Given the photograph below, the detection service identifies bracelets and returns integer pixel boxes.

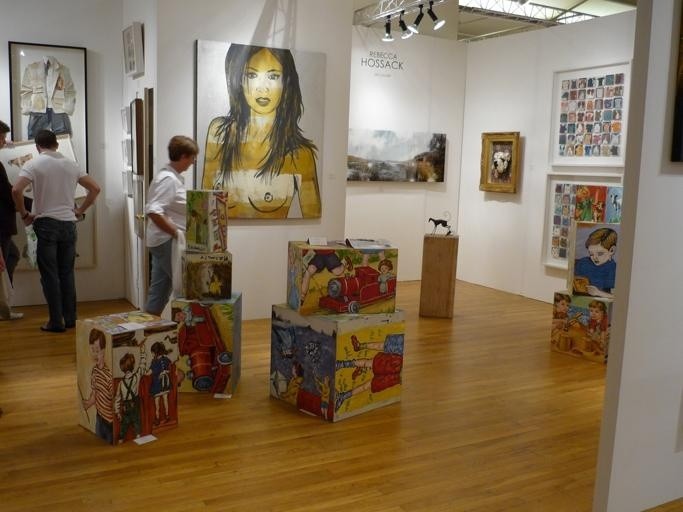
[22,212,29,220]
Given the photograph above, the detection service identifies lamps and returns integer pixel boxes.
[377,1,444,44]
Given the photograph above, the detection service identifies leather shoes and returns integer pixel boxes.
[40,322,65,334]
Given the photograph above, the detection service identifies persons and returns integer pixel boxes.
[301,249,396,306]
[551,292,571,334]
[12,130,100,333]
[335,334,404,411]
[170,306,206,328]
[313,374,331,420]
[81,328,175,445]
[588,300,610,343]
[201,43,321,219]
[0,120,24,321]
[144,136,199,317]
[573,227,618,298]
[278,359,307,406]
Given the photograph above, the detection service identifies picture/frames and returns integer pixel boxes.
[475,129,520,196]
[5,39,88,200]
[118,21,152,241]
[539,171,624,279]
[548,60,634,173]
[4,201,99,273]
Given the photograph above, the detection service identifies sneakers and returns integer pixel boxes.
[3,310,24,320]
